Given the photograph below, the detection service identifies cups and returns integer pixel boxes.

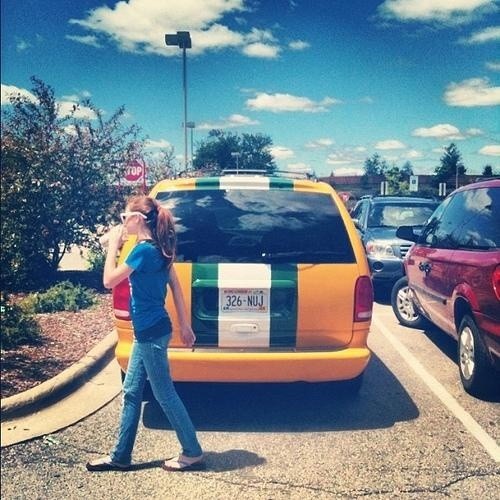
[99,225,120,248]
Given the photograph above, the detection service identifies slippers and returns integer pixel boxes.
[161,458,206,470]
[86,458,130,471]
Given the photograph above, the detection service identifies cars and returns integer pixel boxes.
[109,176,375,394]
[344,182,499,396]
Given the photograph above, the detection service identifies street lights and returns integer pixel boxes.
[165,31,195,177]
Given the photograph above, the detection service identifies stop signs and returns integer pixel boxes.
[123,161,143,182]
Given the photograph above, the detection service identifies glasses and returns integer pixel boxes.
[120,212,148,223]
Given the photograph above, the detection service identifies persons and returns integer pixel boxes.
[86,195,206,472]
